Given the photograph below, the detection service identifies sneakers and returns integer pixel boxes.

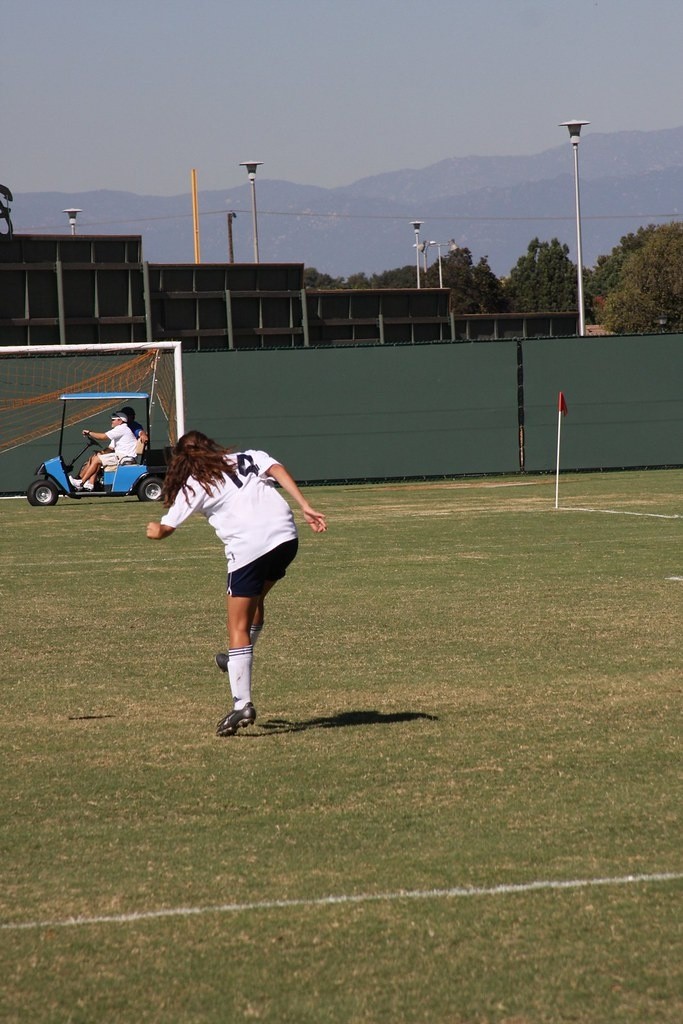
[216,702,255,736]
[215,653,228,671]
[68,475,83,491]
[83,481,94,491]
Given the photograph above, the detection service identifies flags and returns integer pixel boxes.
[558,392,569,417]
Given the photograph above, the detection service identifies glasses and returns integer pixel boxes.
[111,419,115,421]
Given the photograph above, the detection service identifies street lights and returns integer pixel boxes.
[238,160,265,264]
[407,220,424,290]
[412,240,437,288]
[427,240,458,288]
[60,208,82,235]
[558,119,588,336]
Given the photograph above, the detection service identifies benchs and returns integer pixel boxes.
[104,441,148,486]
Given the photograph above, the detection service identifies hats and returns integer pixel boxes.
[110,413,128,422]
[116,407,136,416]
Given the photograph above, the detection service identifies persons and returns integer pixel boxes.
[68,406,149,492]
[146,430,329,737]
